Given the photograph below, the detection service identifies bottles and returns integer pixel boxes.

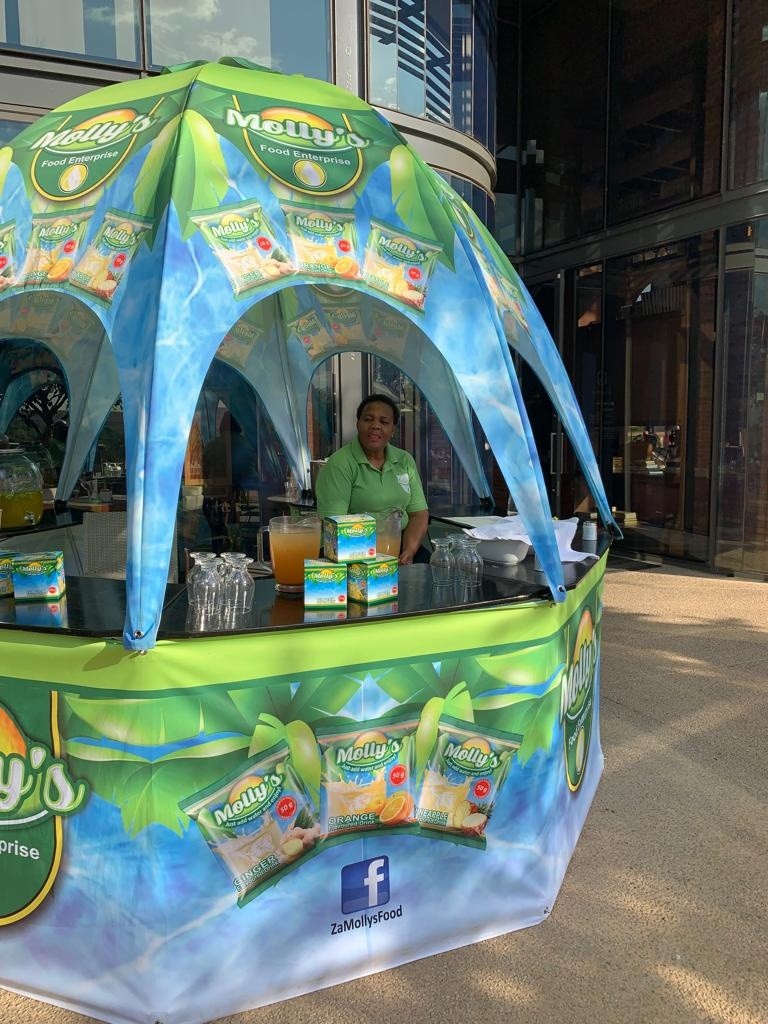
[0,449,43,526]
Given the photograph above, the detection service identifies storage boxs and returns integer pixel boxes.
[0,593,67,628]
[305,601,399,623]
[0,549,66,601]
[323,514,377,563]
[348,553,399,605]
[304,559,347,609]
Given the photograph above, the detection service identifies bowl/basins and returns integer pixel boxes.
[478,540,529,565]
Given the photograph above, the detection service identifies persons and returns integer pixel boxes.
[48,420,68,474]
[315,394,429,565]
[201,411,257,553]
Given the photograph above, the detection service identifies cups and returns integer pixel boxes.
[536,572,548,587]
[582,541,597,554]
[103,462,123,477]
[431,585,484,610]
[534,553,544,572]
[582,521,597,541]
[429,533,484,587]
[184,606,254,634]
[189,551,254,616]
[284,481,295,498]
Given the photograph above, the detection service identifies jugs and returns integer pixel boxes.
[256,516,322,593]
[376,518,401,558]
